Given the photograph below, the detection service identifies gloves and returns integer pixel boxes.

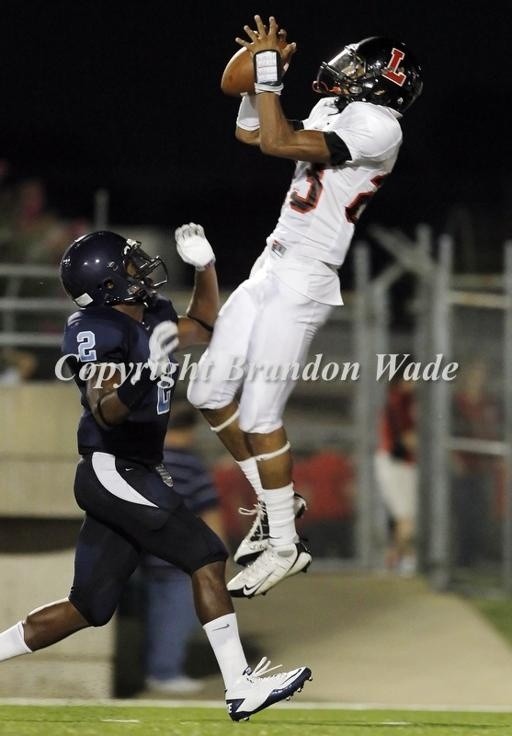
[147,320,179,380]
[174,221,216,272]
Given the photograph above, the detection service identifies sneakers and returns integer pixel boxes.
[234,491,309,567]
[225,539,313,597]
[225,656,313,723]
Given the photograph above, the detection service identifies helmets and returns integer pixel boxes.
[59,230,169,310]
[312,34,424,111]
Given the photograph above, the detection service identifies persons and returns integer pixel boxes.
[184,13,428,596]
[372,353,510,578]
[1,223,313,723]
[1,180,182,384]
[141,397,231,692]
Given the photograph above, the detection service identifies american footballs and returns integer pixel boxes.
[220,40,289,93]
